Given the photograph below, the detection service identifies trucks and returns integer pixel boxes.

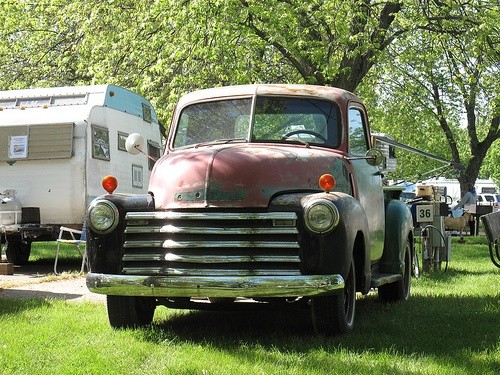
[0,84,164,270]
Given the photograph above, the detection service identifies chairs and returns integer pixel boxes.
[53,220,92,275]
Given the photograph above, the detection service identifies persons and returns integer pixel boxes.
[454,184,479,236]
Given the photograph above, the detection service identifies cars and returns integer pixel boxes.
[82,82,416,339]
[449,193,500,215]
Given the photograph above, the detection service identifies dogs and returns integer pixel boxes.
[444,211,473,242]
